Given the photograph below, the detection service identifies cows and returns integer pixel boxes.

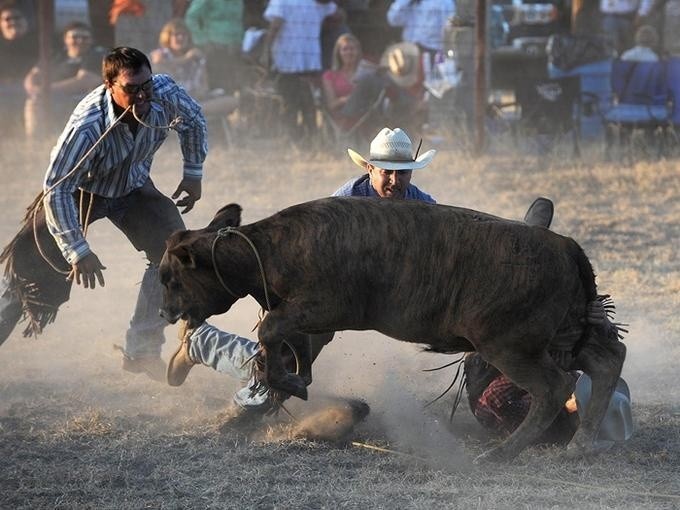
[155,194,627,465]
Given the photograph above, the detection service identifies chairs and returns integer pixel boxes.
[198,54,680,167]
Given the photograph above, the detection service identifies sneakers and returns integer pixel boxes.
[166,318,201,387]
[121,353,169,381]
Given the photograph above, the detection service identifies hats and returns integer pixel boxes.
[377,40,424,90]
[574,368,637,446]
[346,126,438,172]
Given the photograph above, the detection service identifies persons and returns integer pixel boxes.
[463,351,634,446]
[164,126,438,414]
[1,45,207,379]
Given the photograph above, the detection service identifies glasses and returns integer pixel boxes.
[110,76,154,95]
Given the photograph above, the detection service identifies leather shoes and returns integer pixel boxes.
[522,196,555,230]
